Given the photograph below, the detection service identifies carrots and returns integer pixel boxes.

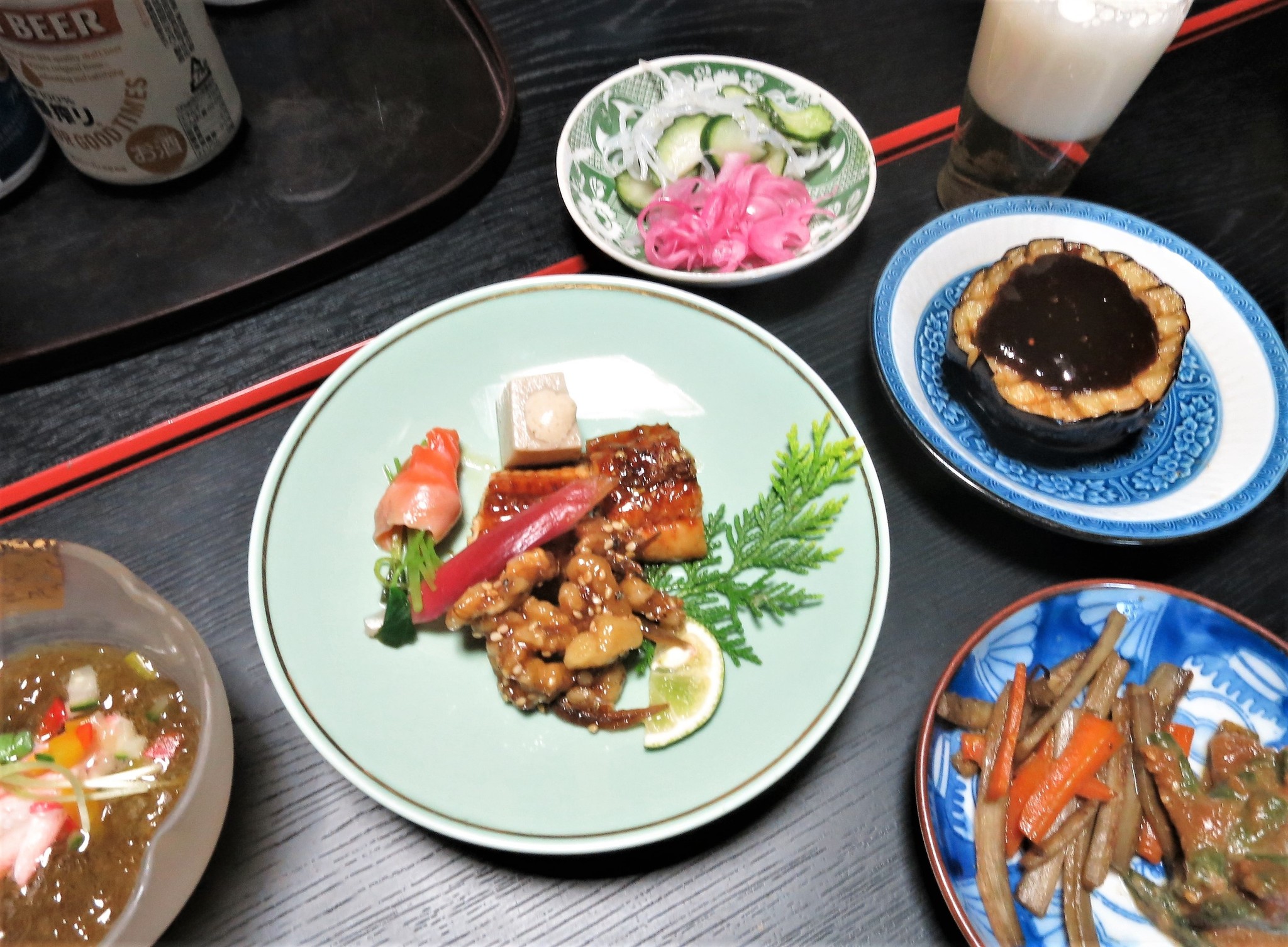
[959,662,1194,862]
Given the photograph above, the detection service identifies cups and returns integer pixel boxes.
[936,1,1192,212]
[0,0,245,185]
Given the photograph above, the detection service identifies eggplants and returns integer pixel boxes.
[943,238,1191,449]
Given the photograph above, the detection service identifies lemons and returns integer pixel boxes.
[643,616,724,750]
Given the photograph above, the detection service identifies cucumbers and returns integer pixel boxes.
[615,87,833,214]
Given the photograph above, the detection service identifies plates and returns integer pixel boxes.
[0,58,48,197]
[555,51,879,289]
[868,194,1288,544]
[249,276,892,854]
[913,577,1288,947]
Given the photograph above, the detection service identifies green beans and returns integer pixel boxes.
[1123,729,1288,947]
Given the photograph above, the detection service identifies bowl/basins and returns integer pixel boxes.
[0,539,235,947]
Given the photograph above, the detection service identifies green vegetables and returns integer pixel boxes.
[372,438,445,648]
